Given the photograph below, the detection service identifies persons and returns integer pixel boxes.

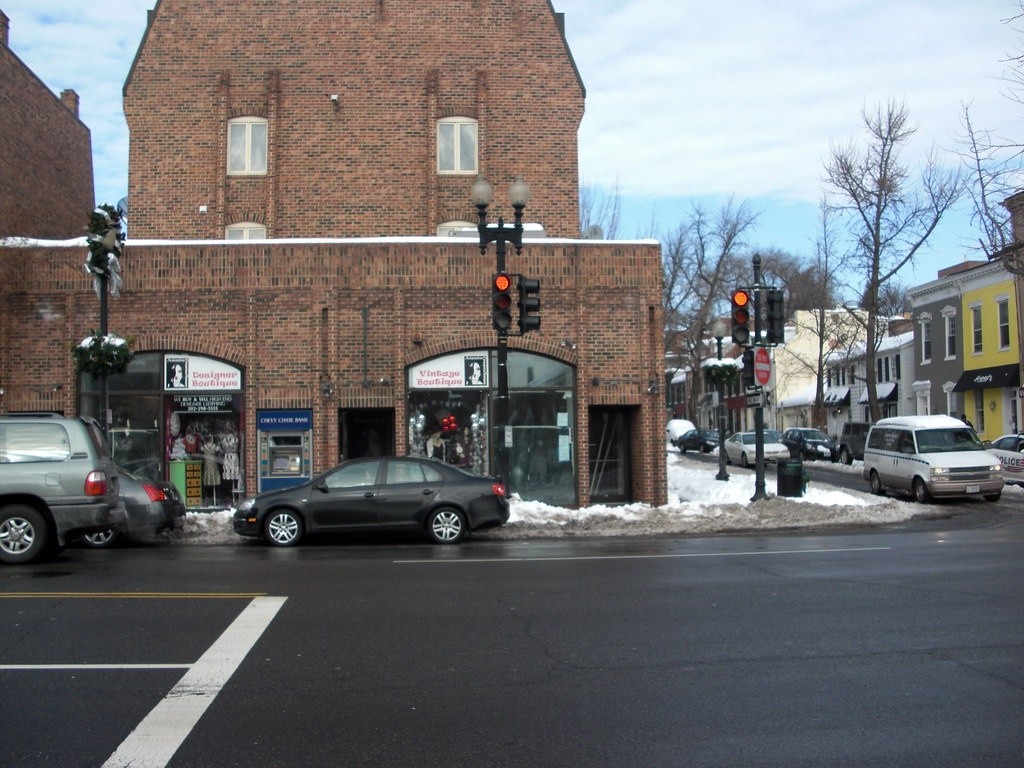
[183,419,240,484]
[426,426,472,467]
[961,414,976,433]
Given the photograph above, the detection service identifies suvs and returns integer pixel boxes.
[0,414,125,565]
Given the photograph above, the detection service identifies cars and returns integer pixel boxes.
[666,419,694,445]
[778,427,838,463]
[984,434,1024,487]
[676,429,720,454]
[76,464,187,551]
[233,455,512,548]
[721,431,791,464]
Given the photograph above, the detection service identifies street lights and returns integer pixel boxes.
[712,318,731,480]
[467,173,530,508]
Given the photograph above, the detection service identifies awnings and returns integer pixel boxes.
[951,363,1021,392]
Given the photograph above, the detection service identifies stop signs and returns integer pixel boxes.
[754,348,772,384]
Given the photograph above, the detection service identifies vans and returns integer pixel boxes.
[863,414,1004,504]
[838,421,876,464]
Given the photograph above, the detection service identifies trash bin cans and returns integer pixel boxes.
[777,459,804,497]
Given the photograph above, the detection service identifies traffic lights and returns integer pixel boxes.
[520,272,542,332]
[492,274,513,334]
[730,289,752,346]
